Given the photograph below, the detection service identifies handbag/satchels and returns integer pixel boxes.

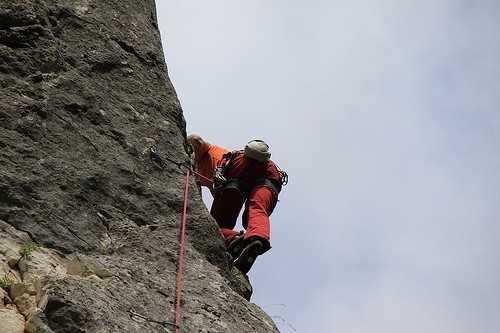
[244,140,271,161]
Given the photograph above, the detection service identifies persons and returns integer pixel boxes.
[187,134,282,275]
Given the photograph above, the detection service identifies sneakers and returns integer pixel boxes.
[225,233,247,260]
[233,240,262,276]
